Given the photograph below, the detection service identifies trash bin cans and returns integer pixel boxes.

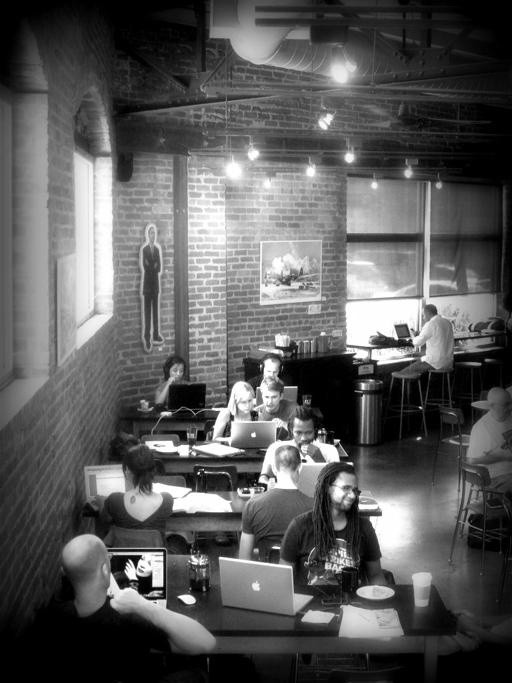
[354,379,385,445]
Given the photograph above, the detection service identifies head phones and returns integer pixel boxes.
[256,353,284,374]
[164,356,180,373]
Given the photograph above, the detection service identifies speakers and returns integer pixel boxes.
[117,151,133,182]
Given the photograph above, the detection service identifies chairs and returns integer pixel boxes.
[445,464,511,578]
[431,403,474,494]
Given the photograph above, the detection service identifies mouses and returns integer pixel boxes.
[176,593,197,605]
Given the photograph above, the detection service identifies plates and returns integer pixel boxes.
[355,584,396,601]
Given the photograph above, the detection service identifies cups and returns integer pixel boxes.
[341,565,357,589]
[303,394,312,405]
[186,427,198,448]
[413,571,432,605]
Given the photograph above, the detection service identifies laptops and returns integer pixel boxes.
[107,547,168,609]
[228,420,277,449]
[296,462,329,498]
[256,385,298,407]
[164,383,206,414]
[83,463,132,501]
[394,324,411,342]
[218,556,315,617]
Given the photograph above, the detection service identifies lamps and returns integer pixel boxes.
[218,46,450,197]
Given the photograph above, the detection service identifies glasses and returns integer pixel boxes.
[330,484,361,496]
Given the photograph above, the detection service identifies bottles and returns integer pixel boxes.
[186,550,211,588]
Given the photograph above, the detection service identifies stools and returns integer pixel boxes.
[346,334,511,433]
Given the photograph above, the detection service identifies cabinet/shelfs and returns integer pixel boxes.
[242,349,358,444]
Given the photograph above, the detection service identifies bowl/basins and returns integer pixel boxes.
[236,485,265,497]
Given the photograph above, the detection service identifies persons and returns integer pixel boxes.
[257,407,340,492]
[437,615,512,682]
[246,354,294,390]
[97,444,172,545]
[144,226,163,349]
[214,381,258,443]
[123,553,155,596]
[237,445,315,562]
[34,532,215,655]
[154,355,190,405]
[277,462,387,585]
[464,386,512,502]
[412,304,453,376]
[257,376,298,423]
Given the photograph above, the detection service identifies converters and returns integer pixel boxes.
[159,411,173,417]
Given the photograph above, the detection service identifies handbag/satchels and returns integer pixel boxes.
[108,432,140,457]
[369,332,398,346]
[467,513,512,552]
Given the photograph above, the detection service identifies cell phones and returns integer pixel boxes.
[411,329,415,333]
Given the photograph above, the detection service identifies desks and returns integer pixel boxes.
[161,581,459,682]
